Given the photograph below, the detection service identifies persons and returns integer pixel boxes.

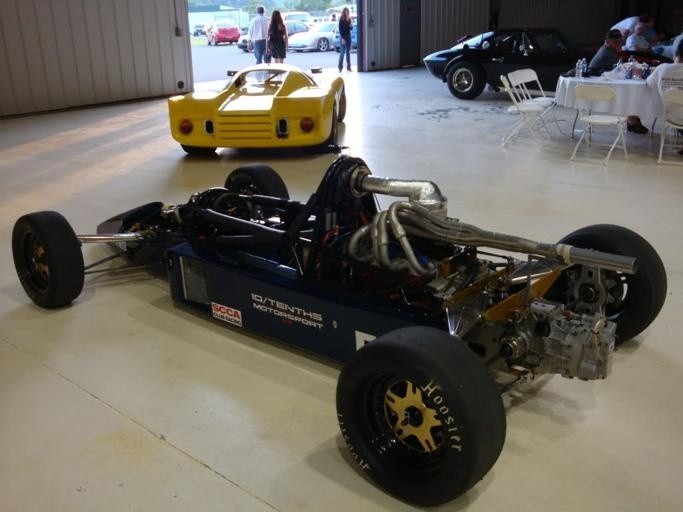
[331,14,337,22]
[264,11,288,63]
[246,6,271,63]
[338,7,353,72]
[586,15,683,160]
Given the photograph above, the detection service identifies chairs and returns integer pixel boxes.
[506,68,566,135]
[657,86,682,165]
[498,75,553,149]
[570,84,626,165]
[267,154,377,277]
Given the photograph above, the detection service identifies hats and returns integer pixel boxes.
[605,29,623,39]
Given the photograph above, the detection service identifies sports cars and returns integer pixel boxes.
[163,60,345,159]
[420,23,672,99]
[8,156,669,511]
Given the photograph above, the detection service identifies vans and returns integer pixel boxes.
[280,11,313,26]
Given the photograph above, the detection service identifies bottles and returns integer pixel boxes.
[616,56,647,80]
[575,58,587,78]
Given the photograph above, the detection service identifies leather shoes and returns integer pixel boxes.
[627,119,648,133]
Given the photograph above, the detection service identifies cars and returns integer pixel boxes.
[192,23,206,37]
[234,21,310,55]
[206,19,239,47]
[287,21,339,53]
[333,16,358,52]
